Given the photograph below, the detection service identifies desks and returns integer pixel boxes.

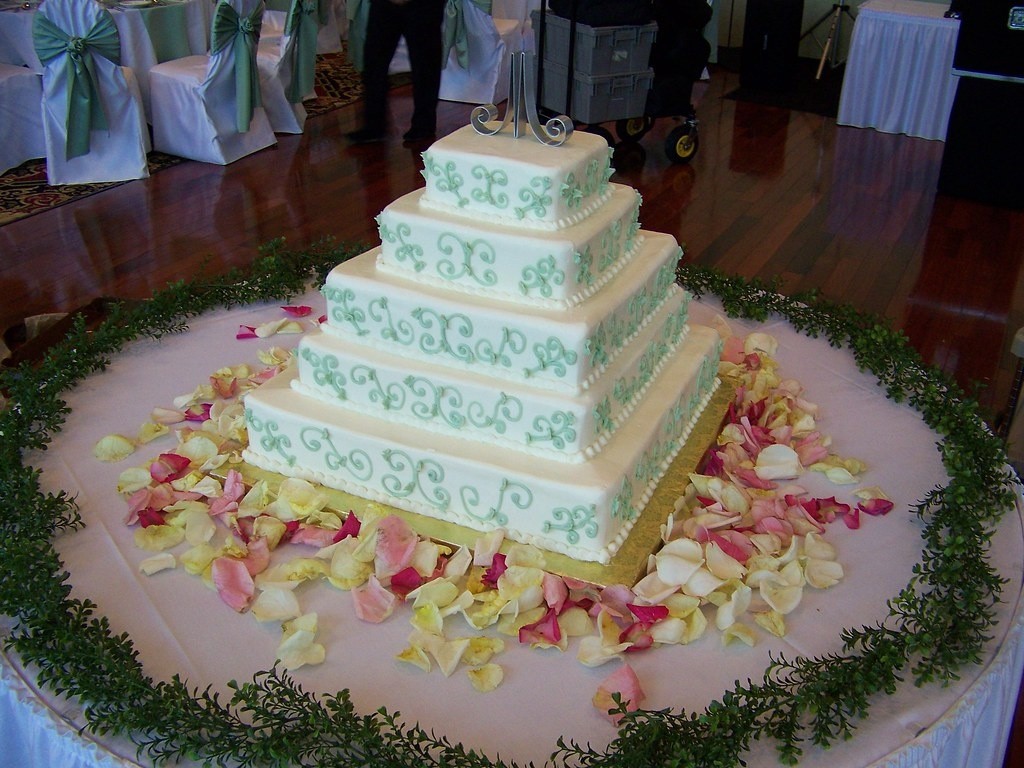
[835,0,962,142]
[0,0,213,128]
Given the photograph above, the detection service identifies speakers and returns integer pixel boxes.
[740,0,804,95]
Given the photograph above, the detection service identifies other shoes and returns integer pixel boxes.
[402,123,437,138]
[345,123,391,140]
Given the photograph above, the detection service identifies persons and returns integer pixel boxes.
[343,0,447,141]
[737,0,806,104]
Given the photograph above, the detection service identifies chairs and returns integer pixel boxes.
[32,0,153,186]
[151,0,279,166]
[346,0,411,76]
[207,0,323,136]
[437,0,524,107]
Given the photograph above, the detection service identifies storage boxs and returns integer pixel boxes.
[529,10,658,76]
[531,54,654,124]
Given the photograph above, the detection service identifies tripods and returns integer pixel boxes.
[800,0,856,81]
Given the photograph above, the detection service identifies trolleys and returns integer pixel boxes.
[531,0,715,165]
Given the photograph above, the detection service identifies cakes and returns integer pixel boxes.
[243,120,722,564]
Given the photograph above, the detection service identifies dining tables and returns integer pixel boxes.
[0,243,1024,768]
